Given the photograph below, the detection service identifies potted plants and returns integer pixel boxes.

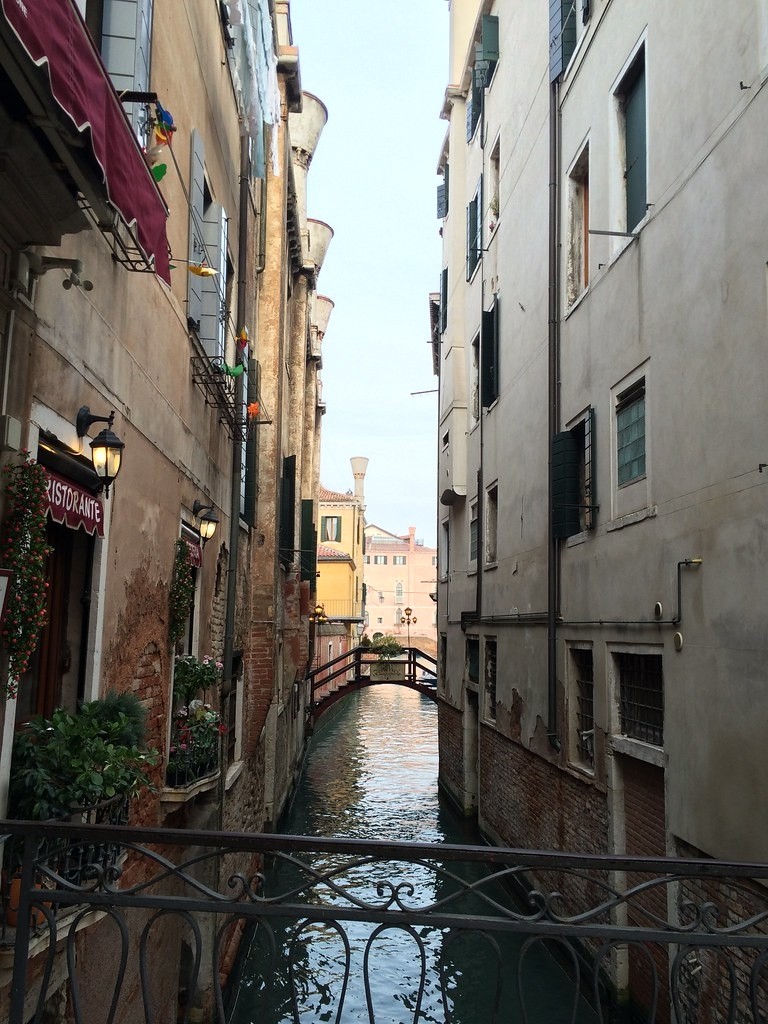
[2,689,157,927]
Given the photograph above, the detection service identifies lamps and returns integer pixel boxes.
[308,605,328,625]
[193,500,220,549]
[76,405,126,500]
[400,607,417,625]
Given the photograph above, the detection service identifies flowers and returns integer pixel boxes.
[167,652,229,785]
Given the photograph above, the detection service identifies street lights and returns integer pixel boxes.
[308,604,329,670]
[400,606,417,681]
